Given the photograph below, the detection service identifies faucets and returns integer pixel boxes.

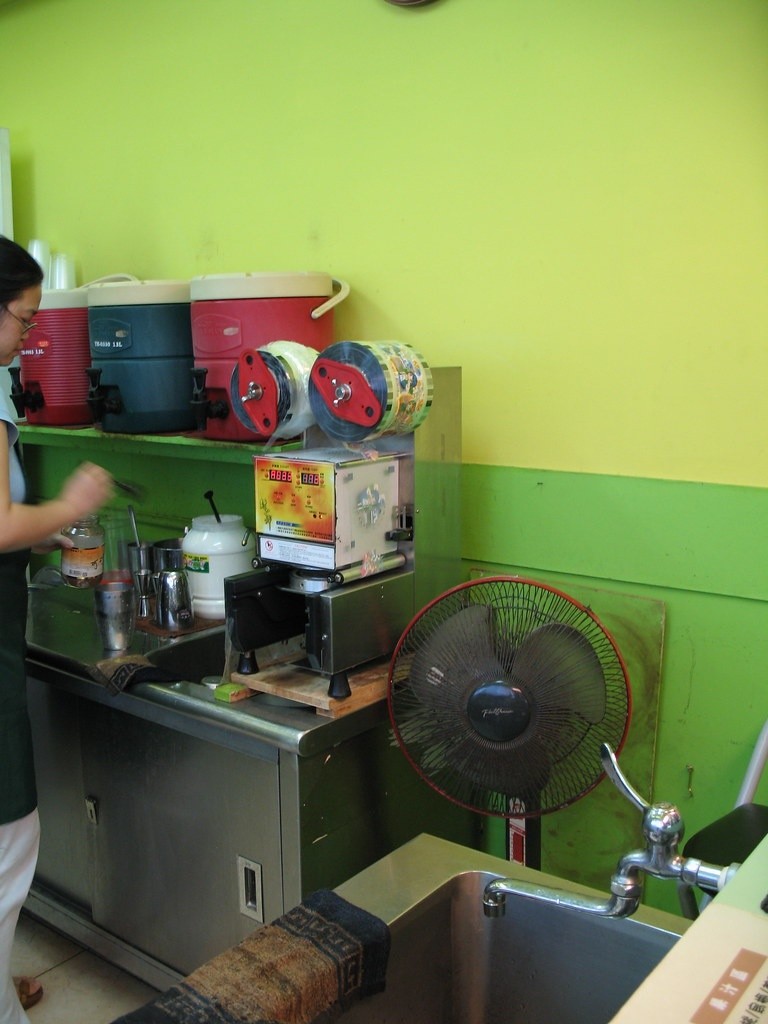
[600,743,738,920]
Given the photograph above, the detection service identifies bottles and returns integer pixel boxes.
[182,513,258,620]
[60,514,105,589]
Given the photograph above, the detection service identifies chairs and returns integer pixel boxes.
[683,717,768,914]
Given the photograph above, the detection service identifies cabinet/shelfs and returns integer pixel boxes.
[22,676,281,995]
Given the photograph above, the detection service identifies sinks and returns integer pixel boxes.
[106,832,699,1024]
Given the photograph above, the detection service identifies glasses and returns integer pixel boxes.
[3,303,38,334]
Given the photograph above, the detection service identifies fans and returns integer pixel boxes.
[386,576,632,870]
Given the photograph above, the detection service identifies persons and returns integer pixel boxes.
[0,236,114,1024]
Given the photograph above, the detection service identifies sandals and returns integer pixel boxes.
[11,974,44,1009]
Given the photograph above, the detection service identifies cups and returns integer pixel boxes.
[94,582,138,651]
[51,252,76,289]
[97,510,143,585]
[28,238,51,290]
[152,537,182,572]
[100,568,134,585]
[151,570,195,630]
[126,541,154,620]
[30,566,70,590]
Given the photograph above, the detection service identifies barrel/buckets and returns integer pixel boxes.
[190,271,353,441]
[7,288,94,425]
[78,273,192,435]
